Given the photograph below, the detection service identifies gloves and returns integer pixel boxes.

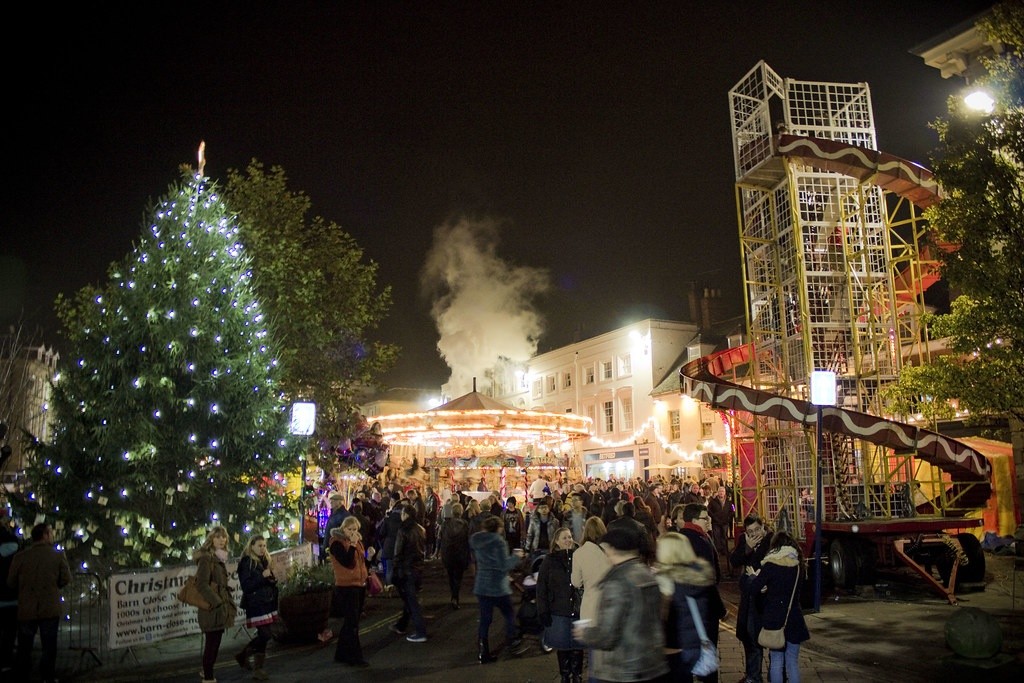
[539,613,553,627]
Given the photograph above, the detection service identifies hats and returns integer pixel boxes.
[330,495,344,500]
[391,493,400,500]
[649,486,658,491]
[508,496,516,505]
[614,500,626,515]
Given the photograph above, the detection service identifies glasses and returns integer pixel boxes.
[698,516,709,521]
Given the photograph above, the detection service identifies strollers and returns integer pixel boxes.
[508,555,553,654]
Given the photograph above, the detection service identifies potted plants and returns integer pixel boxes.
[277,559,333,640]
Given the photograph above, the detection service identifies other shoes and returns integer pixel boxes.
[561,677,570,683]
[199,670,216,683]
[572,675,582,683]
[452,599,462,609]
[335,657,369,668]
[739,675,763,683]
[389,623,406,634]
[406,632,427,642]
[415,585,424,592]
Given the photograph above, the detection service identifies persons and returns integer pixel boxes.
[727,513,811,683]
[407,453,418,475]
[303,477,315,496]
[536,526,584,683]
[178,526,237,683]
[388,504,428,642]
[435,503,475,610]
[322,469,743,542]
[572,529,676,683]
[329,515,371,668]
[655,532,720,683]
[570,516,613,629]
[468,514,531,664]
[8,523,73,683]
[235,535,280,679]
[679,503,731,623]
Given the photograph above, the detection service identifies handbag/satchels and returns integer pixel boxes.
[758,626,786,649]
[180,557,218,610]
[691,641,721,677]
[367,573,383,597]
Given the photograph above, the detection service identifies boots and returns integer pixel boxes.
[509,636,531,655]
[236,647,257,672]
[478,637,498,664]
[430,538,442,560]
[253,654,270,680]
